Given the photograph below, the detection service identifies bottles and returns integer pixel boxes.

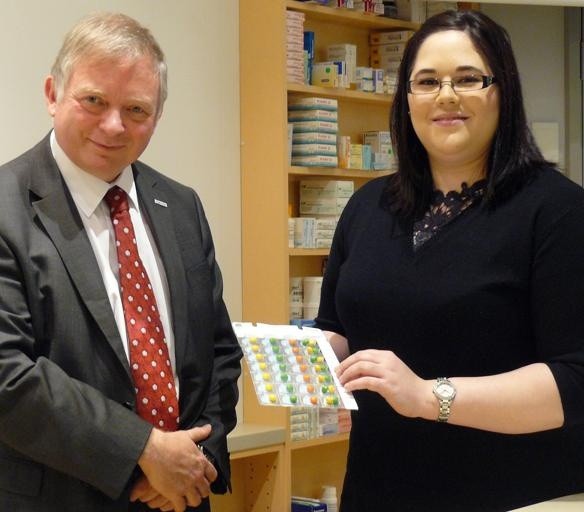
[319,486,337,512]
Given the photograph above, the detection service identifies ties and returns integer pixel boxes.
[105,186,180,432]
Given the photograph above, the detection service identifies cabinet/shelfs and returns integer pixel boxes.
[239,2,483,512]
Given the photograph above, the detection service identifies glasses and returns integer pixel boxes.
[403,74,497,93]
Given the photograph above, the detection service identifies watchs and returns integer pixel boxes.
[433,376,456,424]
[197,445,216,466]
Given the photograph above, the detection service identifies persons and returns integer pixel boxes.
[0,10,243,512]
[313,9,584,512]
